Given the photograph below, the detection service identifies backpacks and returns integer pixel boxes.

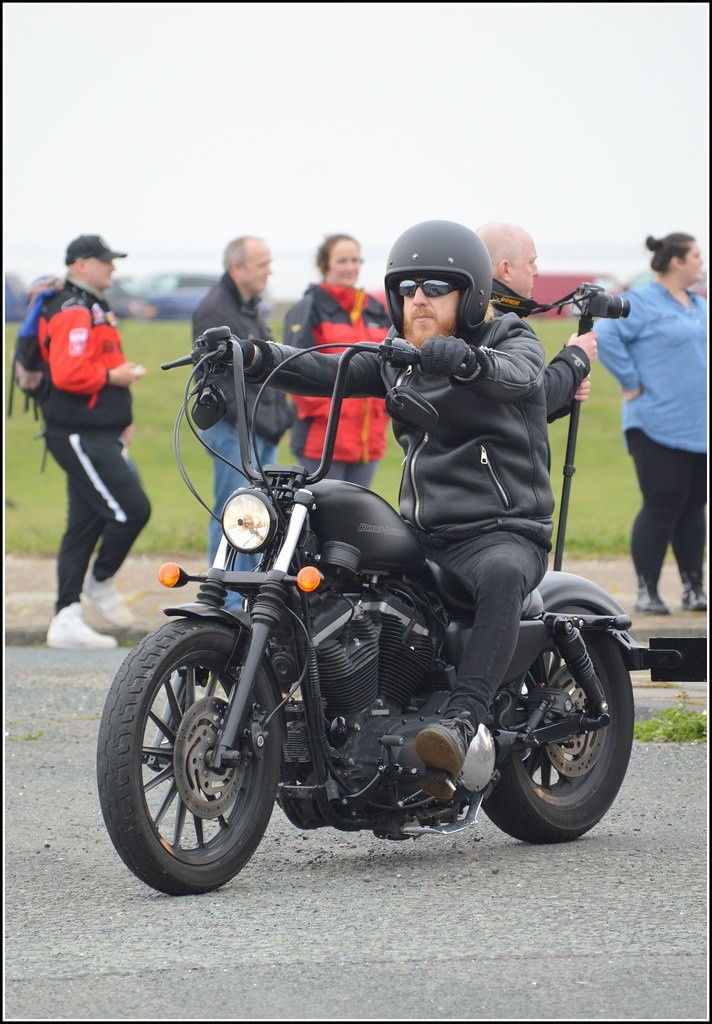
[12,289,94,405]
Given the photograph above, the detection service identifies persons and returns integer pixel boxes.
[192,221,546,799]
[37,226,706,647]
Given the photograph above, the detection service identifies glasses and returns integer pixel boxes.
[398,278,462,298]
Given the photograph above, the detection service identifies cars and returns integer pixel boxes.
[130,273,272,322]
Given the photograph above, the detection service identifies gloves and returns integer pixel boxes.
[192,334,255,374]
[416,335,478,379]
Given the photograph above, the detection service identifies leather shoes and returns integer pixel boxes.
[415,711,475,803]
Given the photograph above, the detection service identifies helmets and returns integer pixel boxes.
[385,220,493,334]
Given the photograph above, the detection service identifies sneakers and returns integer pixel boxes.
[681,584,707,610]
[82,572,135,627]
[46,602,118,651]
[635,587,669,615]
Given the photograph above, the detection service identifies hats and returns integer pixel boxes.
[66,235,127,264]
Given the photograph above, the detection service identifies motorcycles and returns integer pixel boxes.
[96,327,707,894]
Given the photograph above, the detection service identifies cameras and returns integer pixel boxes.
[571,283,630,319]
